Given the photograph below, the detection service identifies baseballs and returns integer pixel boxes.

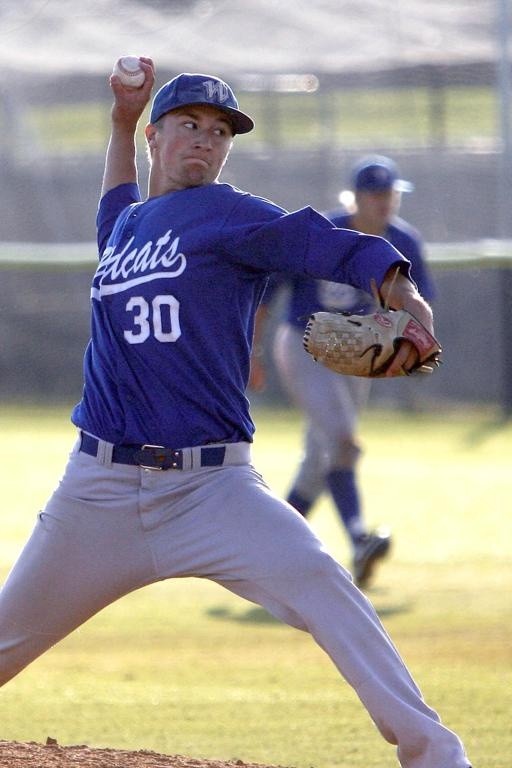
[114,57,149,90]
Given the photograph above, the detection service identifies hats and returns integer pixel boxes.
[150,75,254,136]
[357,165,396,191]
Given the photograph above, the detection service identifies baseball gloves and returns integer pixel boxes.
[303,306,441,379]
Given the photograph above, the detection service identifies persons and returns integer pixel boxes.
[244,154,441,592]
[0,56,471,768]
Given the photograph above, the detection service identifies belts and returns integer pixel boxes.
[79,430,226,469]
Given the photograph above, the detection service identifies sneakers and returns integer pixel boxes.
[353,532,388,585]
